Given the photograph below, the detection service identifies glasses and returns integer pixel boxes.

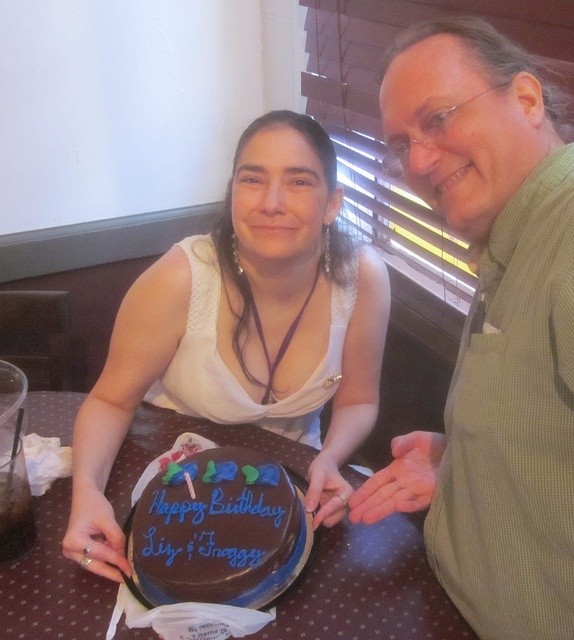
[381,83,510,177]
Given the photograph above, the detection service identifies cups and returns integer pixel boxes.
[1,359,35,560]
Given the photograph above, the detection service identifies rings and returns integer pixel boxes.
[80,560,94,567]
[334,495,349,509]
[81,538,94,557]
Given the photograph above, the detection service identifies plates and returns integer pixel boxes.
[119,466,319,612]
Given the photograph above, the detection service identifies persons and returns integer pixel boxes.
[62,109,390,582]
[348,16,574,640]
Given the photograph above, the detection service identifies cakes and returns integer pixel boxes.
[126,446,306,609]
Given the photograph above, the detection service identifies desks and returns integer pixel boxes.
[0,390,479,640]
